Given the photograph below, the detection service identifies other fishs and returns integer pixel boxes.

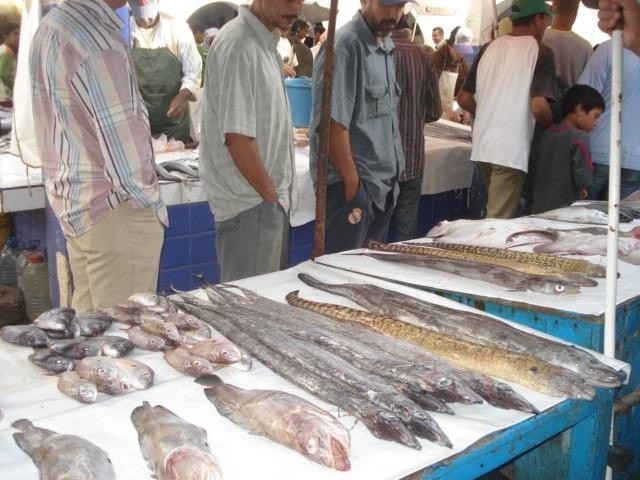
[159,286,422,452]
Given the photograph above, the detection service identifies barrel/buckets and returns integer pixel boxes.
[284,76,312,128]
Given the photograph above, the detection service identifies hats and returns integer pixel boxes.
[127,0,158,19]
[508,0,555,22]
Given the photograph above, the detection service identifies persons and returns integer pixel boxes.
[188,21,210,144]
[432,27,448,50]
[200,0,304,283]
[527,84,606,214]
[310,25,326,60]
[205,27,220,48]
[436,26,473,125]
[0,23,21,108]
[129,0,202,144]
[542,0,594,100]
[577,39,640,201]
[597,0,640,56]
[456,0,558,220]
[307,0,419,255]
[28,0,169,316]
[277,27,299,80]
[387,11,443,242]
[291,19,313,77]
